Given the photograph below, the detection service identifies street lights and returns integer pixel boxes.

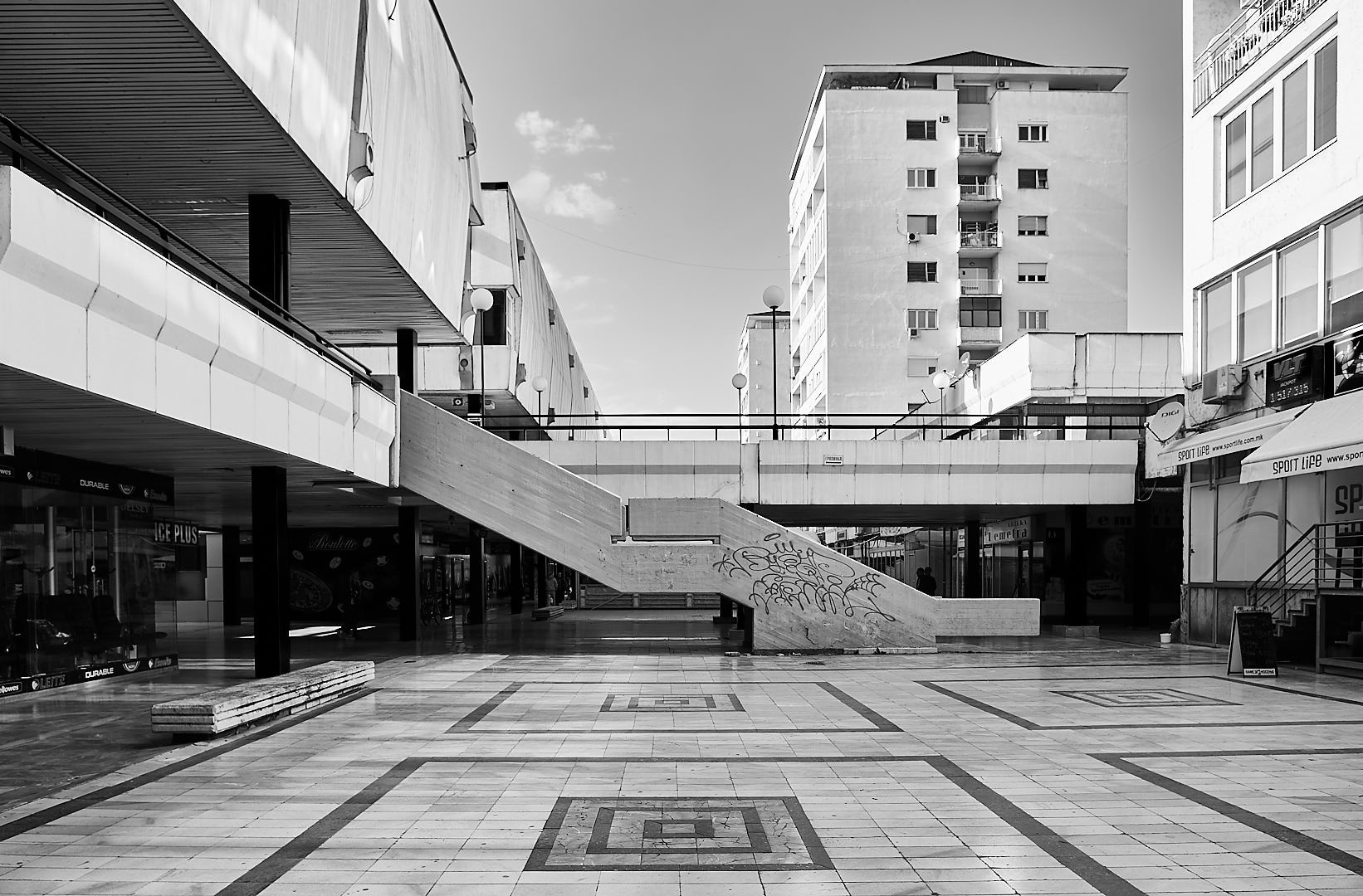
[933,371,951,441]
[763,286,784,440]
[470,286,494,430]
[531,374,546,440]
[731,373,747,442]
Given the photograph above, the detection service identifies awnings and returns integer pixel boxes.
[1157,388,1363,484]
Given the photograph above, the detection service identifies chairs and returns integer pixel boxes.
[12,593,167,667]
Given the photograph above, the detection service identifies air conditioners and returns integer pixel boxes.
[940,116,950,123]
[1202,364,1242,403]
[996,79,1009,90]
[908,328,919,338]
[907,232,920,243]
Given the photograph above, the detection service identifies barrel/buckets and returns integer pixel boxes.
[1160,633,1172,643]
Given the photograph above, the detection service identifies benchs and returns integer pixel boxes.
[532,607,564,620]
[151,661,376,738]
[559,600,577,613]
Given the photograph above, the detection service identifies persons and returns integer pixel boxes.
[916,567,937,597]
[547,571,566,606]
